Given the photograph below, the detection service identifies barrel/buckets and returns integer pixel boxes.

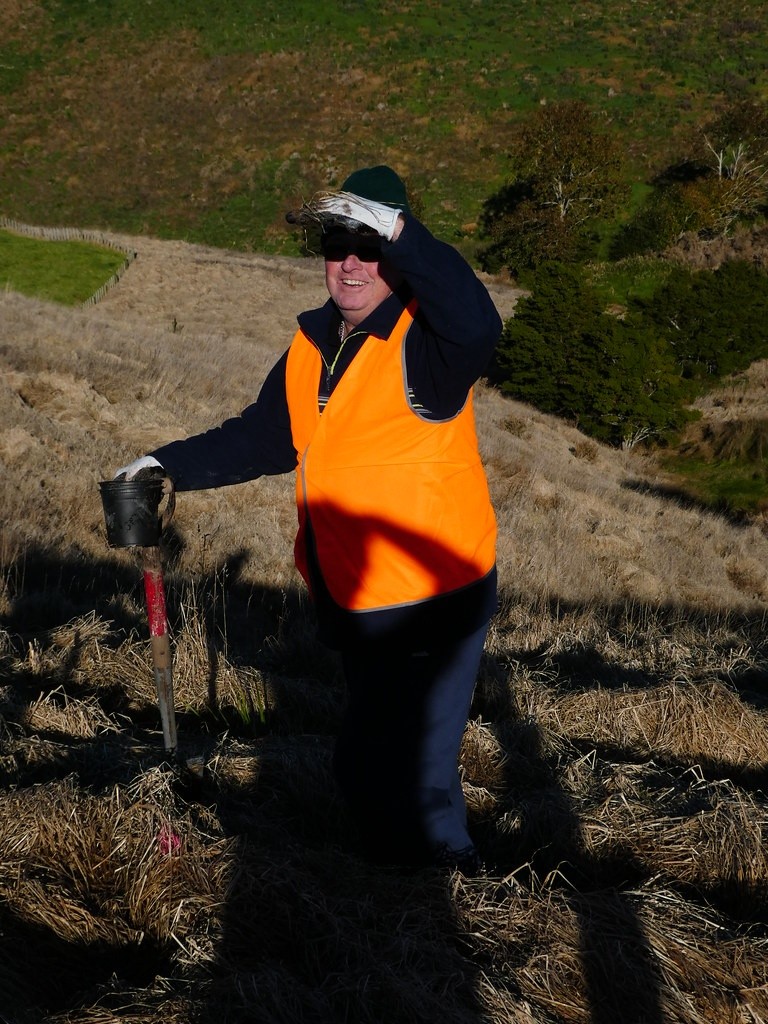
[96,479,165,547]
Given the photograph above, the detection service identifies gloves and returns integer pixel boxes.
[285,190,404,241]
[113,456,165,482]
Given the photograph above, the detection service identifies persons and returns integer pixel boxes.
[115,164,504,895]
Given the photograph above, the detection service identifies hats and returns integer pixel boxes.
[340,164,411,214]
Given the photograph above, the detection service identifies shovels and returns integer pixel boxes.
[107,470,204,797]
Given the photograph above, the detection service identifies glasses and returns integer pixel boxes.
[320,244,385,262]
